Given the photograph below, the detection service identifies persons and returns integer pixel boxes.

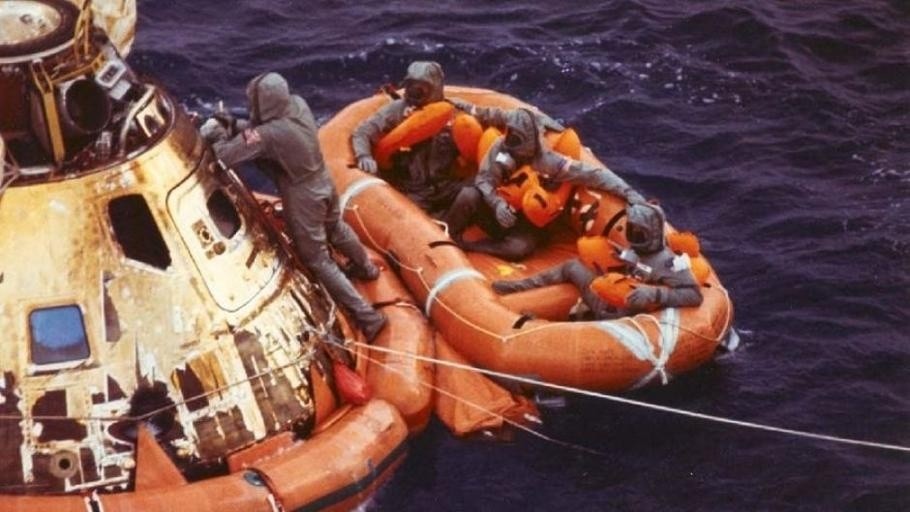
[490,201,703,320]
[202,72,387,344]
[350,62,565,216]
[439,108,647,263]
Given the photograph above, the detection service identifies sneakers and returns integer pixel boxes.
[344,264,379,281]
[364,312,386,342]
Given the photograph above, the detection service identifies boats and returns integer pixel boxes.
[316,86,736,388]
[1,0,436,512]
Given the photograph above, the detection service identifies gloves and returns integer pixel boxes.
[357,155,378,174]
[626,282,668,311]
[495,201,517,229]
[627,190,646,205]
[542,116,565,133]
